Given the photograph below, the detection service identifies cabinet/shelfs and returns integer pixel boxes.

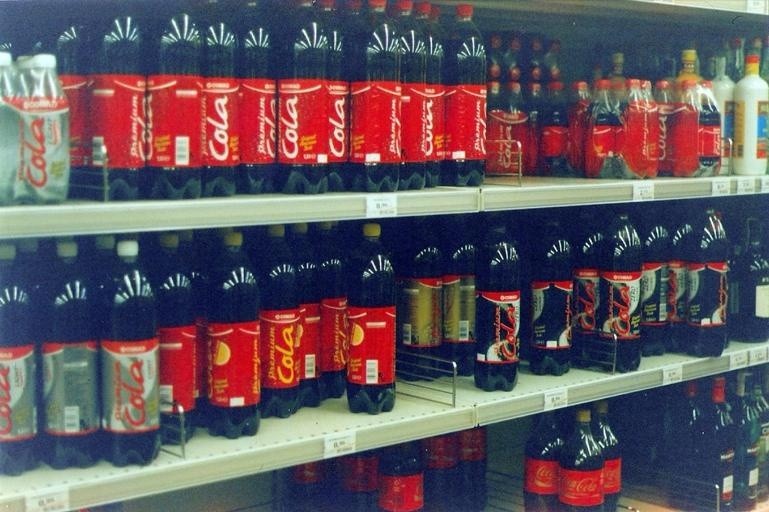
[0,173,768,511]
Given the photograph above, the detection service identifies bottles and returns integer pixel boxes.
[268,369,768,512]
[2,0,769,207]
[2,201,767,480]
